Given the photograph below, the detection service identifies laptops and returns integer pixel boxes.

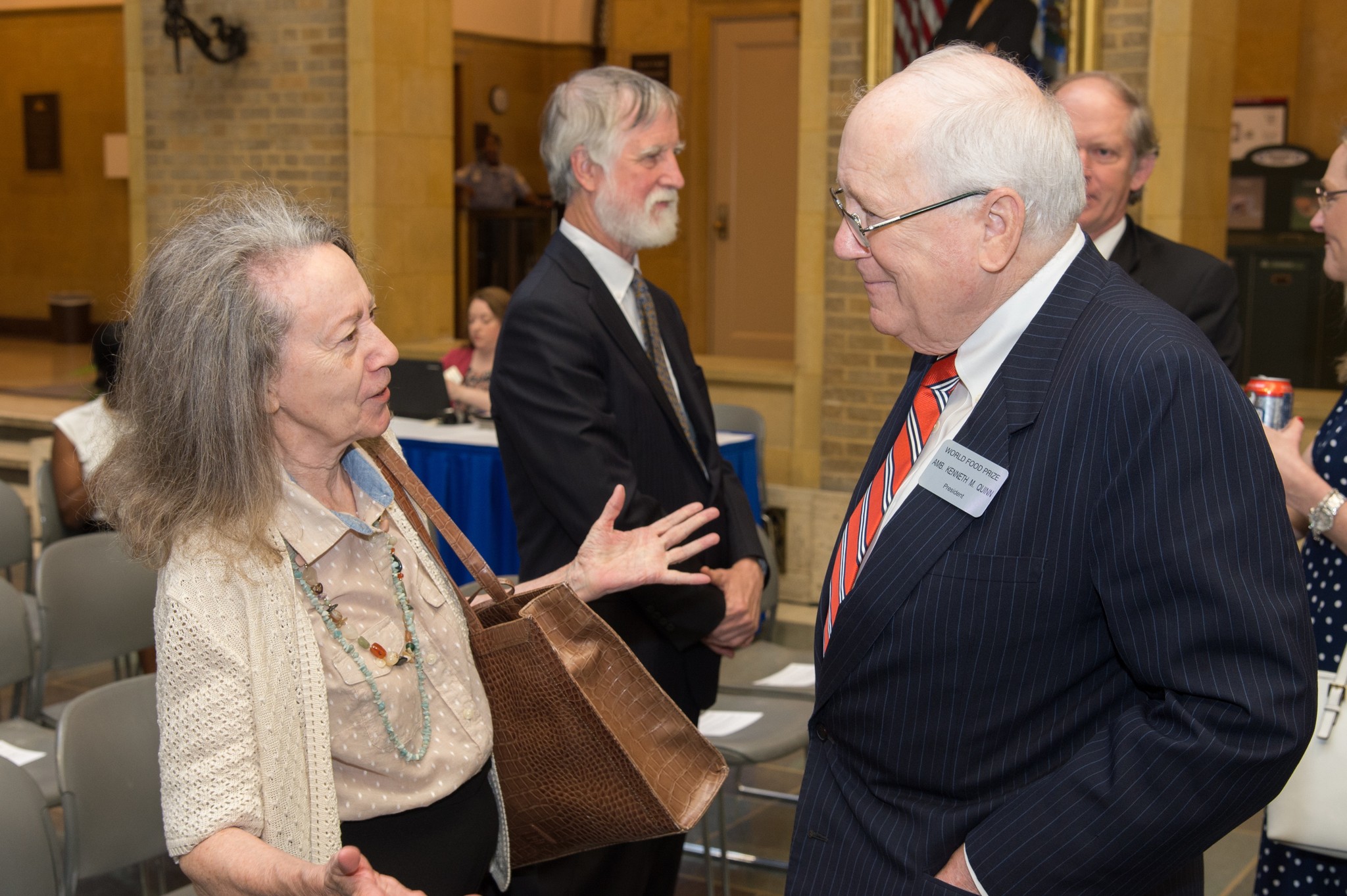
[387,358,461,423]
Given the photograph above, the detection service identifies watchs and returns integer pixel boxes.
[1309,486,1346,543]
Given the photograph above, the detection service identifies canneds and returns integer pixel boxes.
[1244,374,1293,430]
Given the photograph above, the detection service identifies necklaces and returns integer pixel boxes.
[280,514,433,764]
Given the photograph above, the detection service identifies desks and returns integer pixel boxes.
[393,419,762,585]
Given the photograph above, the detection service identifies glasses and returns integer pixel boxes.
[1314,185,1346,211]
[830,188,993,247]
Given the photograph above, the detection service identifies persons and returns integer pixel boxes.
[454,133,551,289]
[1043,69,1248,388]
[785,42,1321,896]
[492,67,773,895]
[1251,142,1347,895]
[91,186,719,896]
[50,323,127,536]
[439,287,511,415]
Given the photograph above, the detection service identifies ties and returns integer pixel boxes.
[822,350,962,657]
[631,268,701,461]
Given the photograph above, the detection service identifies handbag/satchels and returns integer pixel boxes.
[359,432,729,870]
[1263,644,1347,860]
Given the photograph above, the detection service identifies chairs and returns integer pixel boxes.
[0,460,199,896]
[684,406,815,896]
